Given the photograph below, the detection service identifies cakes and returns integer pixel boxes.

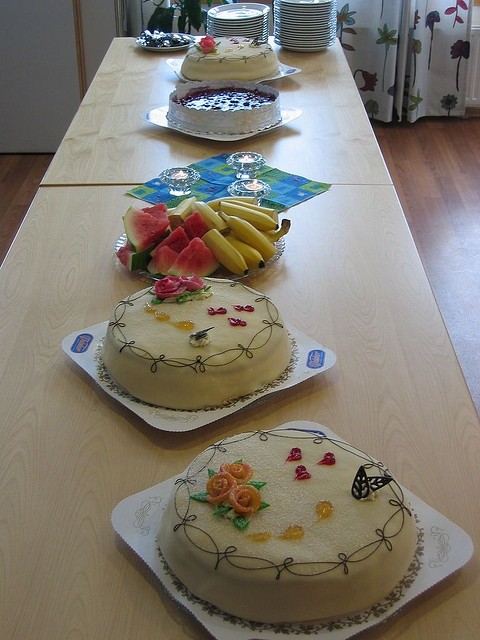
[181,35,280,80]
[166,80,283,135]
[99,275,291,409]
[154,427,419,622]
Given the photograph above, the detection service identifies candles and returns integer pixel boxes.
[225,179,271,207]
[156,166,201,196]
[224,151,268,179]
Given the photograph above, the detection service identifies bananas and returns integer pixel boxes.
[171,196,290,277]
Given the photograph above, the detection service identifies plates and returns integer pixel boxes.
[274,0,337,53]
[134,32,196,52]
[113,207,285,282]
[206,3,270,45]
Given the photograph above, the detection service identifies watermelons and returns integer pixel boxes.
[118,202,219,277]
